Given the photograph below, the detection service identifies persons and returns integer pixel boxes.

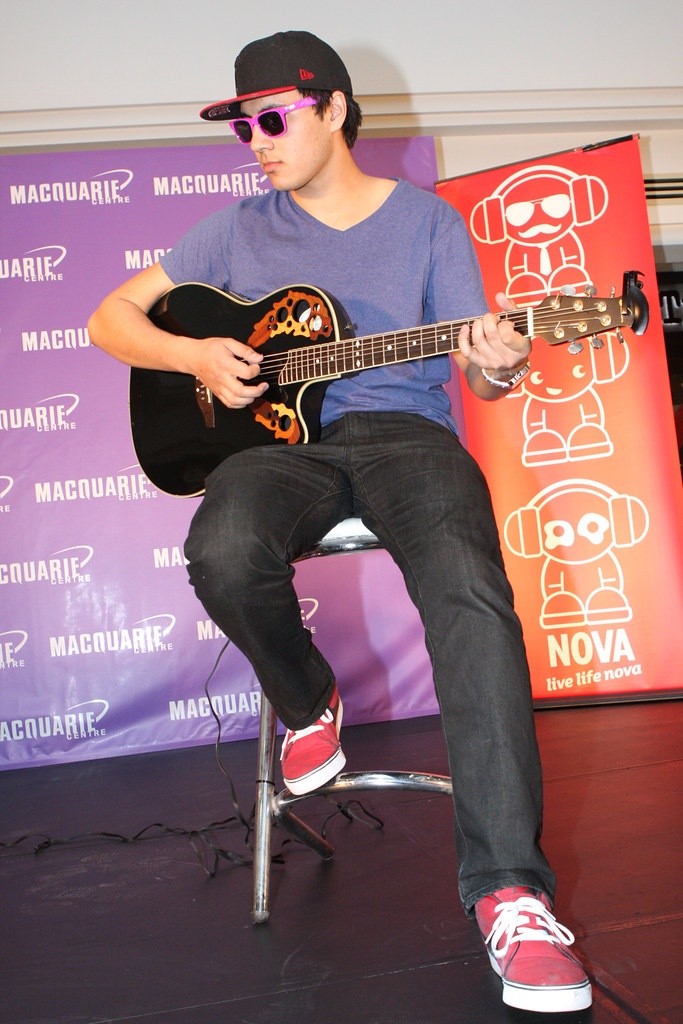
[86,31,593,1012]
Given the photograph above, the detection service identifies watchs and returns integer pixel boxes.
[481,362,532,388]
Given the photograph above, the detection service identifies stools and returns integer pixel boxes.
[250,516,454,922]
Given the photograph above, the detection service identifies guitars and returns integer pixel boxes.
[127,269,651,500]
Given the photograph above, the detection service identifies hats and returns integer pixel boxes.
[198,30,353,120]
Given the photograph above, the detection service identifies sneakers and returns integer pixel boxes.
[279,675,347,796]
[472,885,593,1013]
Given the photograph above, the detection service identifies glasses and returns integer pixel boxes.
[229,94,322,144]
